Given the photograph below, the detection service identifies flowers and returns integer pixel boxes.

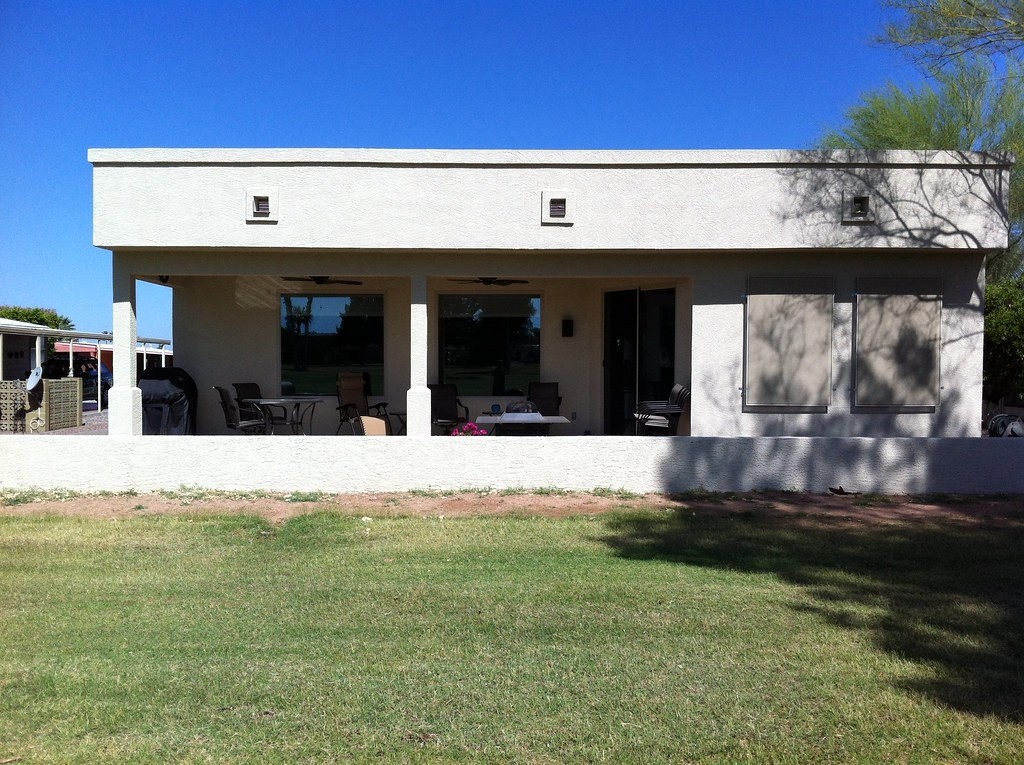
[451,422,487,436]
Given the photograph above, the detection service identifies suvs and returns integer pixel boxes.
[17,355,113,407]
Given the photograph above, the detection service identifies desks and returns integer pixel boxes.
[389,412,406,435]
[233,398,324,435]
[475,416,571,436]
[482,411,505,436]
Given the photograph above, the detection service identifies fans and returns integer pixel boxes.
[447,277,530,286]
[280,276,363,286]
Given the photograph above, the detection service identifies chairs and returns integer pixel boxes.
[232,383,288,435]
[213,386,274,435]
[631,383,690,436]
[336,372,393,435]
[526,382,562,416]
[427,384,469,436]
